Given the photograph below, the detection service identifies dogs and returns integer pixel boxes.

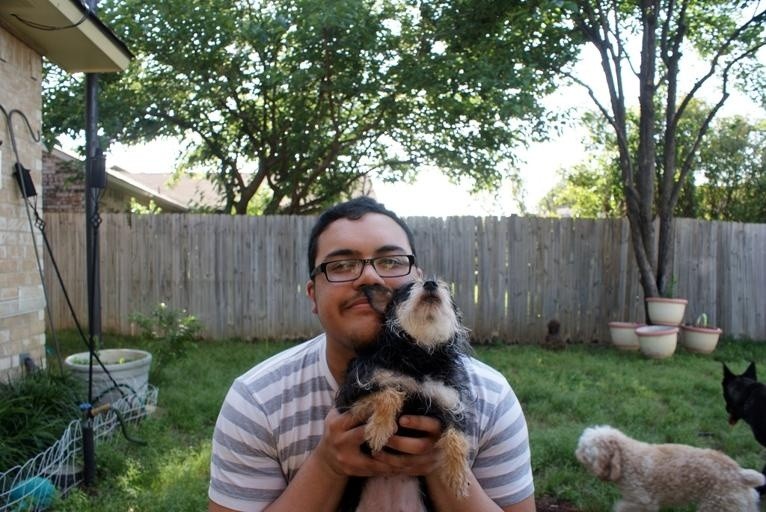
[573,423,766,512]
[333,272,478,512]
[720,360,766,494]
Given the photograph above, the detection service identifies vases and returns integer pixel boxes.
[606,321,678,360]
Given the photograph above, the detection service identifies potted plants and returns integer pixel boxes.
[645,270,689,326]
[65,348,153,414]
[680,313,723,354]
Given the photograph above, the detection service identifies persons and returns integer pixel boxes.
[208,196,537,512]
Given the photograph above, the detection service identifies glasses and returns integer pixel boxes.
[310,255,414,282]
